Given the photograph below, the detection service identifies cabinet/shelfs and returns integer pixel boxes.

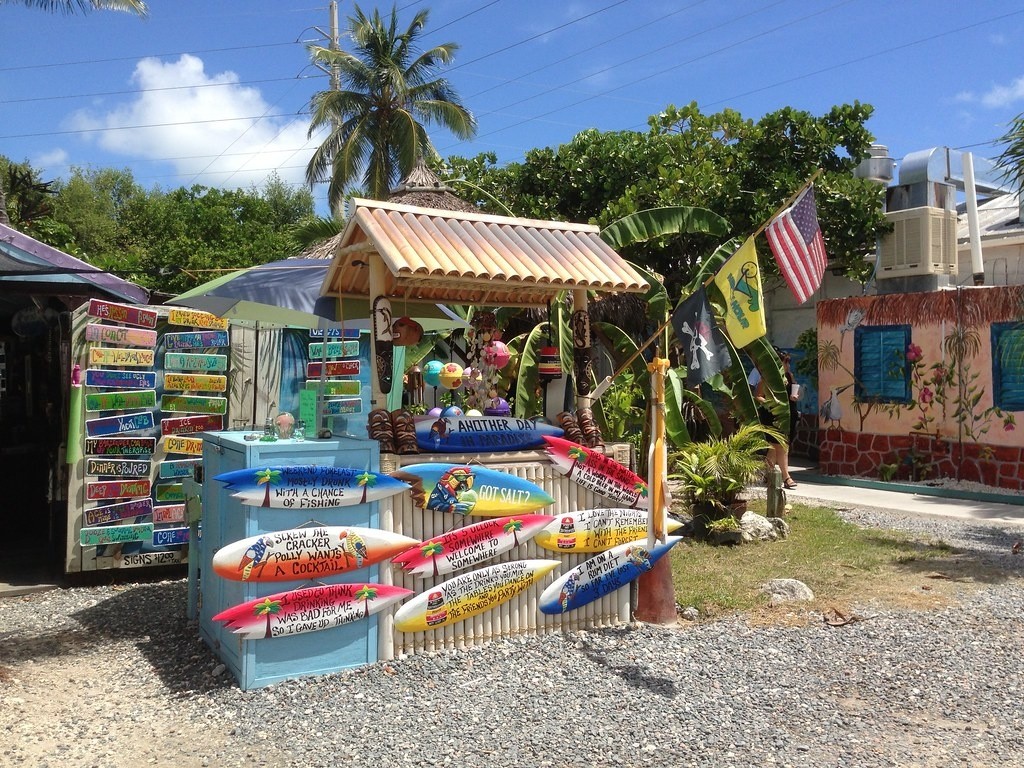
[202,427,378,690]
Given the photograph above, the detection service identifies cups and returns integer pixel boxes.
[295,428,305,442]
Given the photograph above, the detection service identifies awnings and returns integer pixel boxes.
[0,223,152,304]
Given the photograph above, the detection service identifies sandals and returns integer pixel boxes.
[784,477,797,487]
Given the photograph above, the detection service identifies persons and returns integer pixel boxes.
[747,345,800,490]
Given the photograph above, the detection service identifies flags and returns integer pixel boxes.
[713,235,768,351]
[671,284,732,392]
[762,180,830,306]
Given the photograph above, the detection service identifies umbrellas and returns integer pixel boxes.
[164,256,473,438]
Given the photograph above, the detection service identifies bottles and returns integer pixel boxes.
[264,418,274,436]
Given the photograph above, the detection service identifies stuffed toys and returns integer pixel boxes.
[392,316,424,347]
